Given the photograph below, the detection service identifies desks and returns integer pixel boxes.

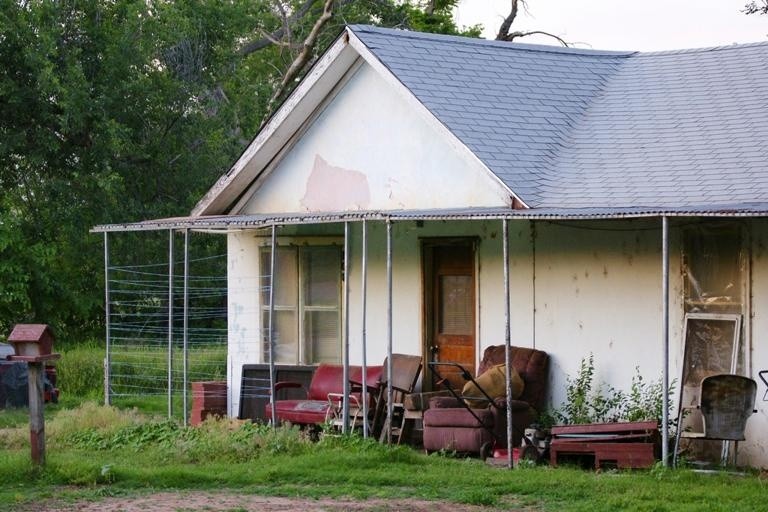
[394,408,426,445]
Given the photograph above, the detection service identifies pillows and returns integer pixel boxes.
[459,361,524,411]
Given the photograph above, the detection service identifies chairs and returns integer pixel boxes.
[338,350,425,445]
[420,343,549,463]
[671,372,759,478]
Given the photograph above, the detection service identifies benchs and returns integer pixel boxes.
[262,360,386,438]
